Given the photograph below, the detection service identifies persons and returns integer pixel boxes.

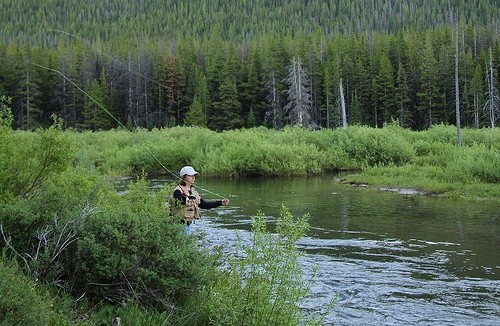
[168,165,230,228]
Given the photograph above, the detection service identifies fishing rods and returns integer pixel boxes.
[29,63,228,240]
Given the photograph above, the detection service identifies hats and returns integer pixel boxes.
[180,166,199,176]
